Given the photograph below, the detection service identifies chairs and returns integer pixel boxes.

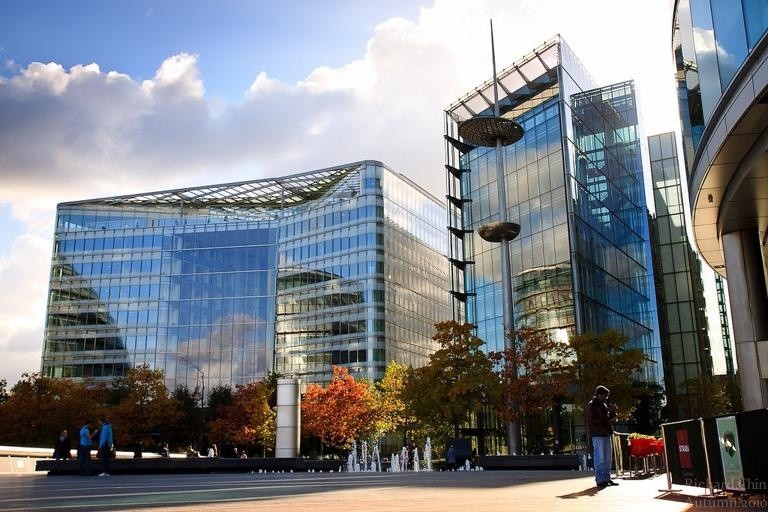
[628,438,665,478]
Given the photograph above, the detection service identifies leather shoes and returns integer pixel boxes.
[597,480,619,488]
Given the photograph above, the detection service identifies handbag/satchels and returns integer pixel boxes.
[96,448,116,458]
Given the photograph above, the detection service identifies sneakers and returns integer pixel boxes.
[99,472,110,477]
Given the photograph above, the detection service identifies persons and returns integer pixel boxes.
[96,415,116,477]
[131,438,248,459]
[77,420,97,477]
[445,443,457,470]
[52,429,71,460]
[584,385,620,489]
